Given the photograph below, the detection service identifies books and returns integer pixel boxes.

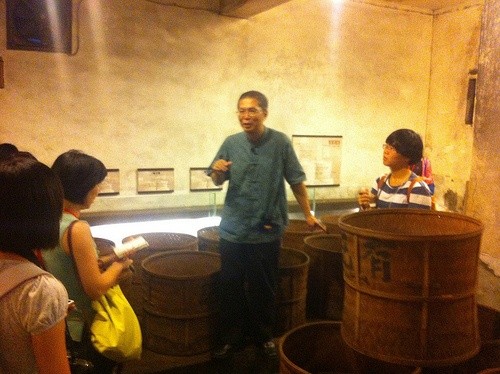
[113,237,149,258]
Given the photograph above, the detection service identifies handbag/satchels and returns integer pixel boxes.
[67,219,144,363]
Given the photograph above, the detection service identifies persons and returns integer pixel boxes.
[0,144,70,374]
[51,151,134,374]
[207,91,327,356]
[359,129,432,211]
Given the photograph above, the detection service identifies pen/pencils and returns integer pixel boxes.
[129,265,135,272]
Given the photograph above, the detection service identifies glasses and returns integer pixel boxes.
[383,143,397,154]
[237,107,265,117]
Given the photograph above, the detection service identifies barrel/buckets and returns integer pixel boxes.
[94,207,500,374]
